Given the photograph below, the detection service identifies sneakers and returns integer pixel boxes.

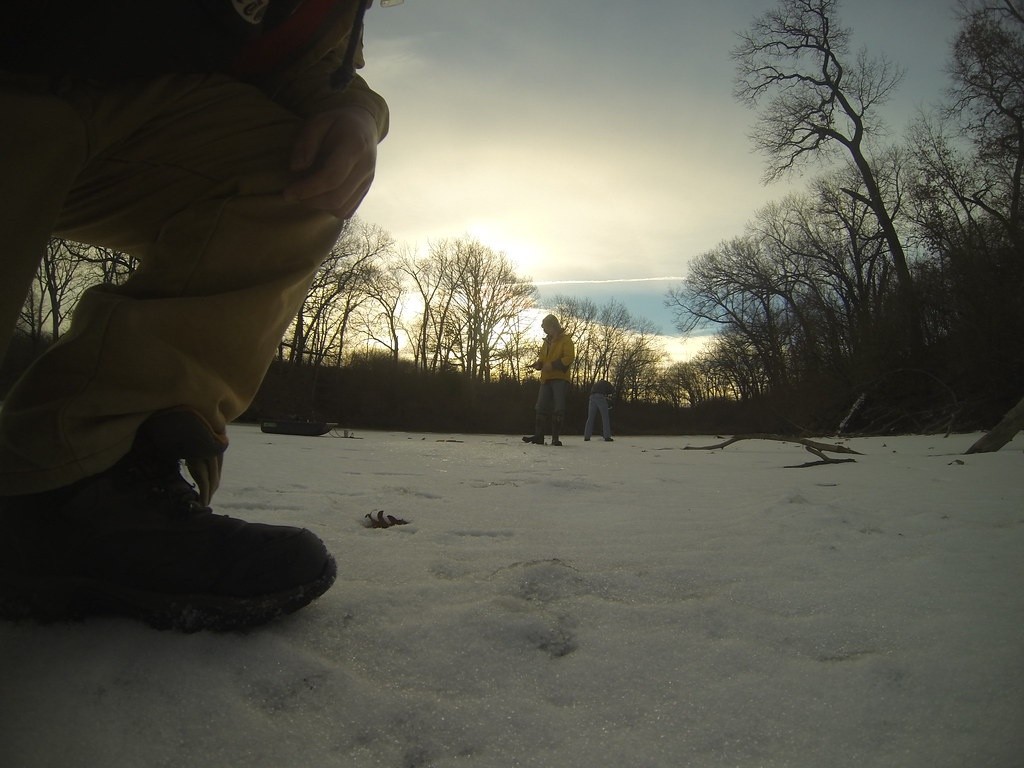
[0,434,335,632]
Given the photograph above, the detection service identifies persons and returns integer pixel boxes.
[522,314,575,446]
[0,0,391,639]
[584,380,616,442]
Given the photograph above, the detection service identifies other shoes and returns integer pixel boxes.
[584,438,590,441]
[605,437,613,441]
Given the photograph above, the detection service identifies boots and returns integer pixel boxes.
[522,414,545,445]
[552,414,561,447]
